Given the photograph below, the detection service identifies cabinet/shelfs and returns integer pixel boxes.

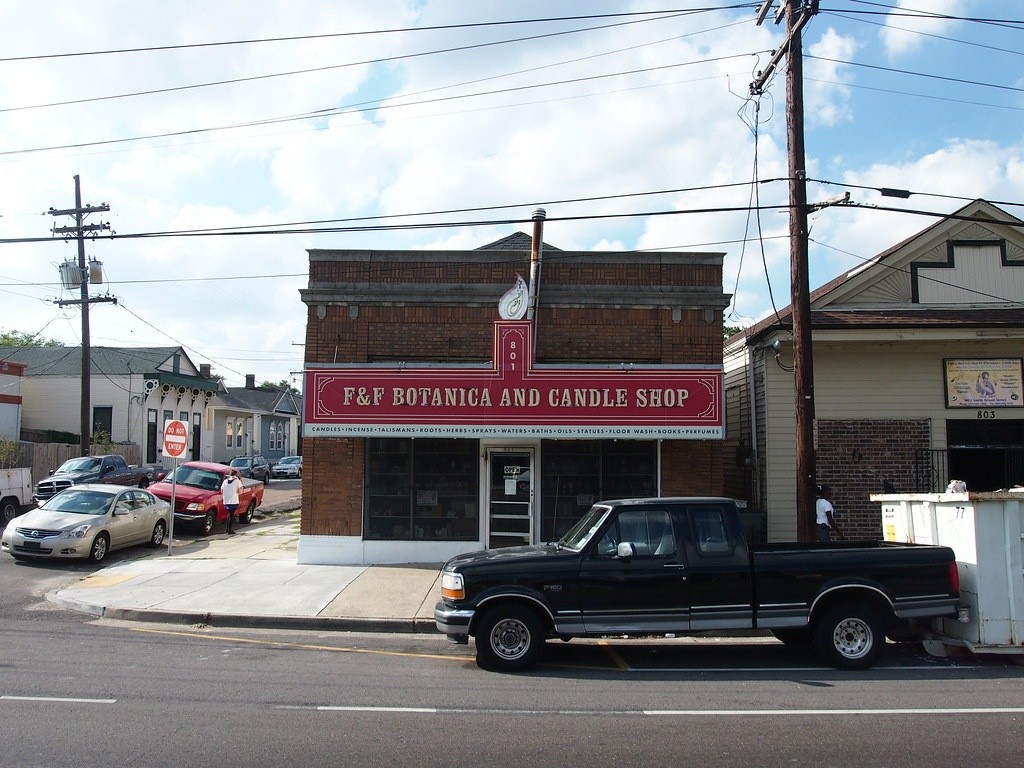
[366,439,477,540]
[541,439,656,541]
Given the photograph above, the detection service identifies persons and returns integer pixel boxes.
[88,498,108,515]
[138,471,154,489]
[815,485,844,542]
[221,470,243,534]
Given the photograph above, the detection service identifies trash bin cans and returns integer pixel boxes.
[868,491,1024,655]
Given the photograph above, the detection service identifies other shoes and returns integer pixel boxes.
[225,530,235,534]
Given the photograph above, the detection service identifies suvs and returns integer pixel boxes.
[230,456,270,487]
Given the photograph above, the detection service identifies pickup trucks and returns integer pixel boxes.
[147,461,264,535]
[34,453,156,504]
[431,429,962,672]
[0,467,34,525]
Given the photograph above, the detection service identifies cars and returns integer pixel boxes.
[3,482,173,564]
[271,455,303,479]
